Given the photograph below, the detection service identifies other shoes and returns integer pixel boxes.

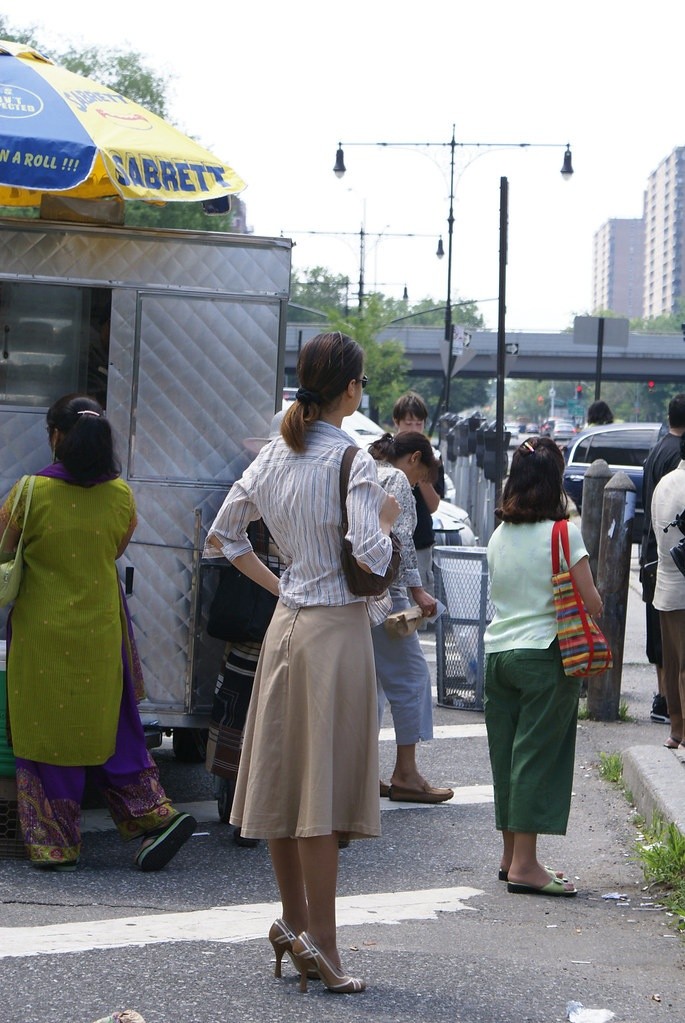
[650,694,670,722]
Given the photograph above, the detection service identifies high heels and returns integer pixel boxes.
[270,918,323,980]
[293,931,364,992]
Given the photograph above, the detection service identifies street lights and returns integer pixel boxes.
[279,229,445,325]
[287,278,410,322]
[334,122,575,417]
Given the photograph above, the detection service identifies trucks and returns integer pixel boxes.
[0,217,292,764]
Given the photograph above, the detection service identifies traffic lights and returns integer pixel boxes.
[648,378,656,392]
[576,385,583,399]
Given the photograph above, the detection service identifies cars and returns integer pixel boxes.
[278,387,480,552]
[558,423,672,547]
[538,419,557,438]
[506,420,540,438]
[554,423,577,441]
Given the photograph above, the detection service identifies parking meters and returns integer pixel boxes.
[438,406,508,552]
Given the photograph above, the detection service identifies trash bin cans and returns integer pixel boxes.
[431,545,497,712]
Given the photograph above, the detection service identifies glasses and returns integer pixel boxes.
[346,375,368,389]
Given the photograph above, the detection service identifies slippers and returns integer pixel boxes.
[136,812,197,872]
[498,868,570,882]
[390,781,454,803]
[507,876,579,895]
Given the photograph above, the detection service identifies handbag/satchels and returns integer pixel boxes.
[207,565,285,643]
[551,519,615,677]
[0,475,35,605]
[338,446,401,595]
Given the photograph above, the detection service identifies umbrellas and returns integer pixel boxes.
[0,39,247,217]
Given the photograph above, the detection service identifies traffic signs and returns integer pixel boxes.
[447,326,473,348]
[504,342,520,357]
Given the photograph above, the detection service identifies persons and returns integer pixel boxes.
[368,390,455,804]
[484,437,602,897]
[0,393,198,873]
[587,400,614,427]
[202,333,400,992]
[639,394,685,749]
